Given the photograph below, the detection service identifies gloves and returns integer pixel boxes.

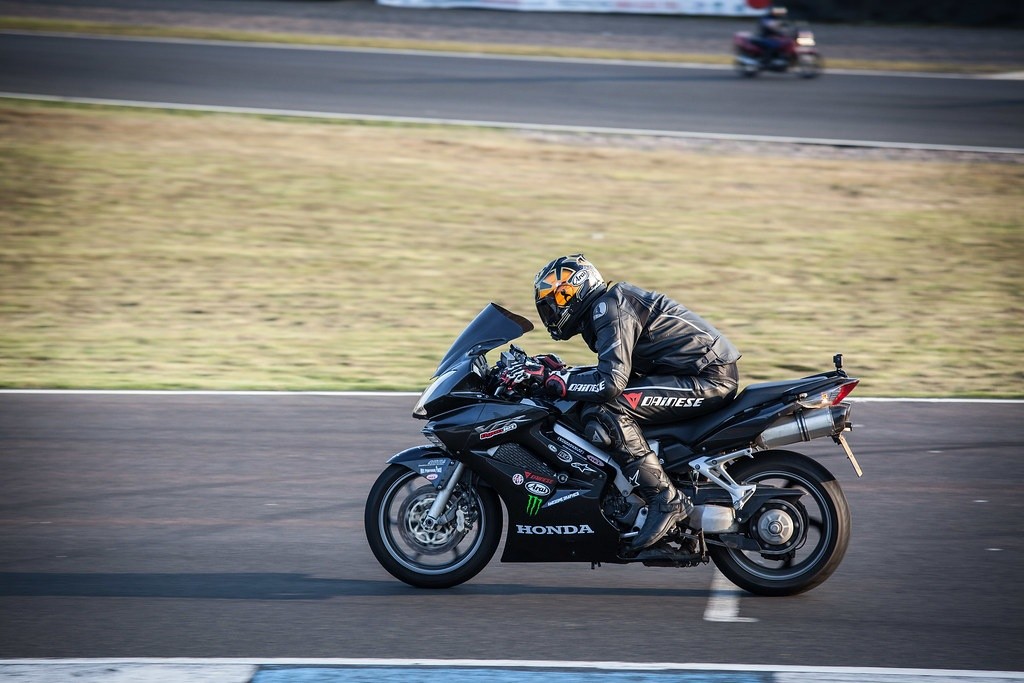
[502,361,571,399]
[531,353,573,373]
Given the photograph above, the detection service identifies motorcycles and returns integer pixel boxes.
[731,21,825,80]
[363,300,863,598]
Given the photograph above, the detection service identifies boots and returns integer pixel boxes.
[622,451,694,549]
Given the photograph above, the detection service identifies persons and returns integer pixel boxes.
[758,6,798,72]
[498,252,742,548]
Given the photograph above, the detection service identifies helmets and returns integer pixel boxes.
[534,254,606,340]
